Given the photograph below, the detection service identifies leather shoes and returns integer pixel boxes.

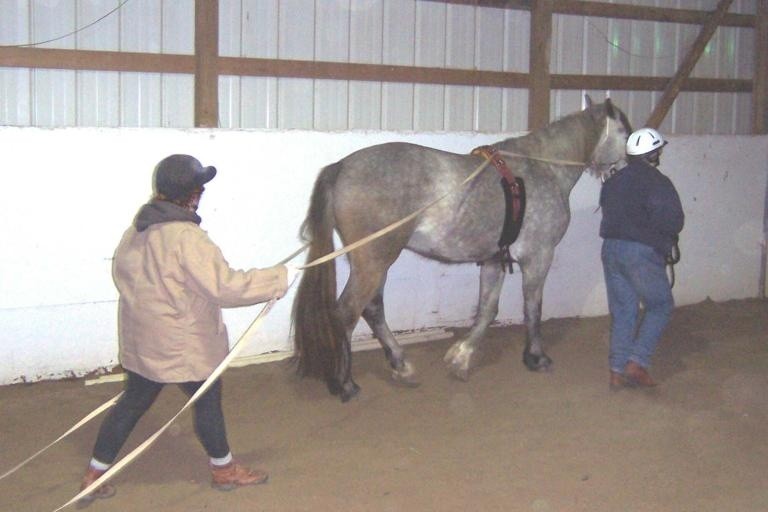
[609,359,658,391]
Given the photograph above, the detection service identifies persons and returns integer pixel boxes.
[78,154,298,500]
[599,126,682,388]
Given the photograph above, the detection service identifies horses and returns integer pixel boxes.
[279,91,635,405]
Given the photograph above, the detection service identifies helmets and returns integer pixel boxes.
[626,128,668,160]
[151,155,216,196]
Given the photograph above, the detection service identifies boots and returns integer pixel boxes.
[81,458,116,500]
[207,458,269,491]
